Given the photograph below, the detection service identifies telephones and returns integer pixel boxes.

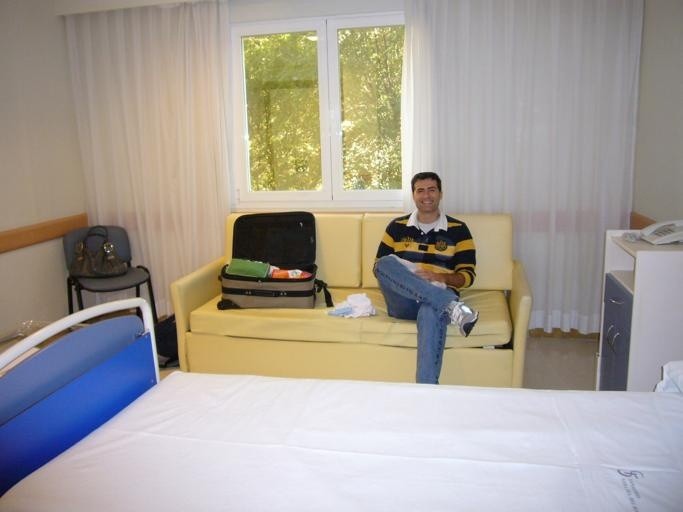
[637,218,683,248]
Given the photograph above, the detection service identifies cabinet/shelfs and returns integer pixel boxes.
[594,228,683,391]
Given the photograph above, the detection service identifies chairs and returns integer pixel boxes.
[63,225,159,333]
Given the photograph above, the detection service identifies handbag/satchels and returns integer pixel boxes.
[71,226,126,277]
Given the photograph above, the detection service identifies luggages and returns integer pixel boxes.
[218,212,333,310]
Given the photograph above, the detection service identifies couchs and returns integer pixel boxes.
[167,212,534,388]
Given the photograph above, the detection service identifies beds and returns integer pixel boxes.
[0,296,683,512]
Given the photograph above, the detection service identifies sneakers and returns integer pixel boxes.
[451,302,479,337]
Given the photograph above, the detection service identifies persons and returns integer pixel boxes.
[370,172,481,385]
